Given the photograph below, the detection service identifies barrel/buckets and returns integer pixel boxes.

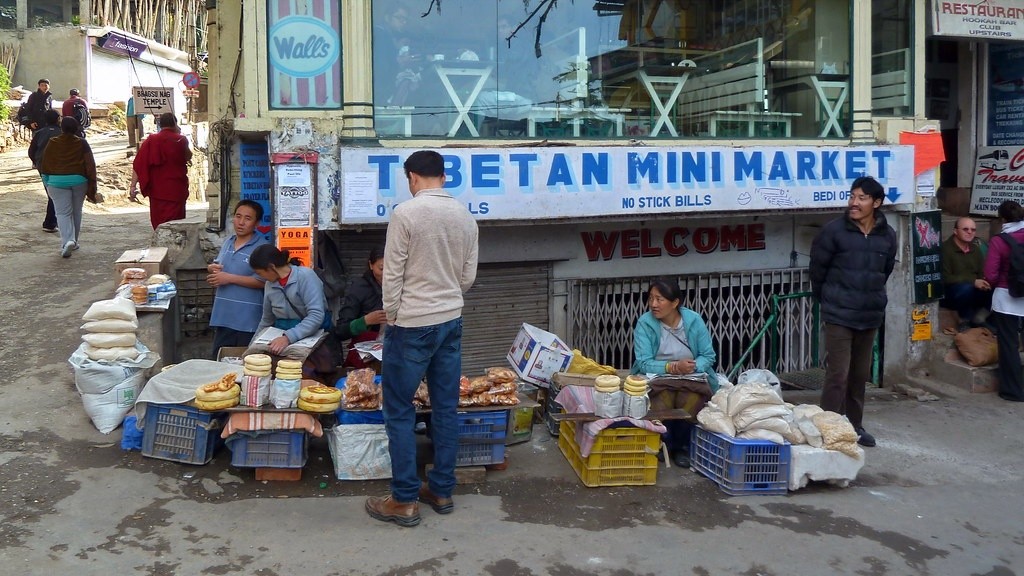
[505,383,538,446]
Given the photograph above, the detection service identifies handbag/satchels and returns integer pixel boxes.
[16,102,32,130]
[954,327,998,366]
[308,328,343,375]
[73,97,91,128]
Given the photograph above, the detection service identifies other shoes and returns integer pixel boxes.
[62,241,75,257]
[127,146,133,148]
[979,322,997,335]
[73,244,79,251]
[42,227,59,231]
[657,452,665,462]
[674,454,690,467]
[956,319,970,332]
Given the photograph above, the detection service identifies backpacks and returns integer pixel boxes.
[992,233,1024,297]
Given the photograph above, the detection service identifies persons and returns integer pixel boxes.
[248,244,342,385]
[984,201,1024,402]
[808,176,896,447]
[366,150,479,527]
[940,217,1003,334]
[206,200,269,361]
[631,280,719,468]
[26,77,97,258]
[130,113,193,230]
[154,114,161,133]
[371,2,543,135]
[126,87,145,148]
[336,244,389,369]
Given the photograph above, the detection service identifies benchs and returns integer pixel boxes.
[842,48,912,137]
[673,39,803,134]
[375,104,415,136]
[527,27,632,138]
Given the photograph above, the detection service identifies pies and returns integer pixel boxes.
[594,374,648,395]
[194,354,342,412]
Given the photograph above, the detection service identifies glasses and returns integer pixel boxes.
[957,228,976,232]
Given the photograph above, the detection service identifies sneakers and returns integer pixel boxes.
[418,482,454,514]
[855,427,876,446]
[365,496,421,526]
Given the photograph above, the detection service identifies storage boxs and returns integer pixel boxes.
[216,346,248,363]
[545,378,567,436]
[334,374,387,480]
[690,425,791,495]
[141,401,223,464]
[114,246,167,285]
[558,409,659,487]
[231,413,306,468]
[507,322,573,388]
[452,411,507,466]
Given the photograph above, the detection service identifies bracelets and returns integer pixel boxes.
[666,363,671,372]
[130,185,136,187]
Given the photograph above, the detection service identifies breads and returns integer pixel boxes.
[119,267,148,304]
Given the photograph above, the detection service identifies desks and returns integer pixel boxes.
[767,74,850,137]
[604,64,706,138]
[423,58,503,139]
[136,294,177,378]
